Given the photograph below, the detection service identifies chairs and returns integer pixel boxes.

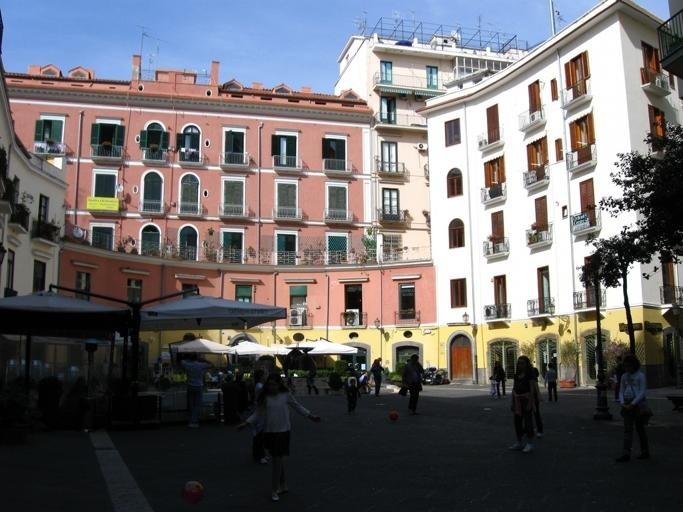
[254,454,271,466]
[614,449,650,462]
[270,487,288,502]
[506,430,544,453]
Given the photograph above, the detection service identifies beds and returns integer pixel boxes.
[176,338,235,355]
[0,291,131,343]
[270,344,292,355]
[307,340,358,357]
[232,341,275,357]
[289,341,315,349]
[138,293,287,332]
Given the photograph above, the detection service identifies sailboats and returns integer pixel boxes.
[389,410,400,420]
[182,481,205,503]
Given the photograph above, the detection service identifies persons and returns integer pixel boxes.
[153,357,163,391]
[369,359,384,396]
[218,370,257,426]
[614,356,626,402]
[251,368,271,466]
[346,377,361,416]
[614,353,653,463]
[487,375,499,400]
[544,363,558,403]
[359,371,369,393]
[403,358,410,386]
[531,367,546,437]
[493,361,506,399]
[507,354,535,452]
[304,355,320,396]
[234,372,321,500]
[181,357,212,414]
[406,353,424,415]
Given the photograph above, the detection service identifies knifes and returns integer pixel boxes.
[289,308,306,326]
[538,230,548,241]
[529,111,540,123]
[346,308,360,326]
[494,242,504,253]
[478,140,486,147]
[484,306,496,319]
[417,143,428,150]
[655,79,668,89]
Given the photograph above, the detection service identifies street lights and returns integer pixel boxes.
[637,402,654,425]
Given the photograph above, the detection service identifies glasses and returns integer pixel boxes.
[374,318,381,329]
[462,311,471,326]
